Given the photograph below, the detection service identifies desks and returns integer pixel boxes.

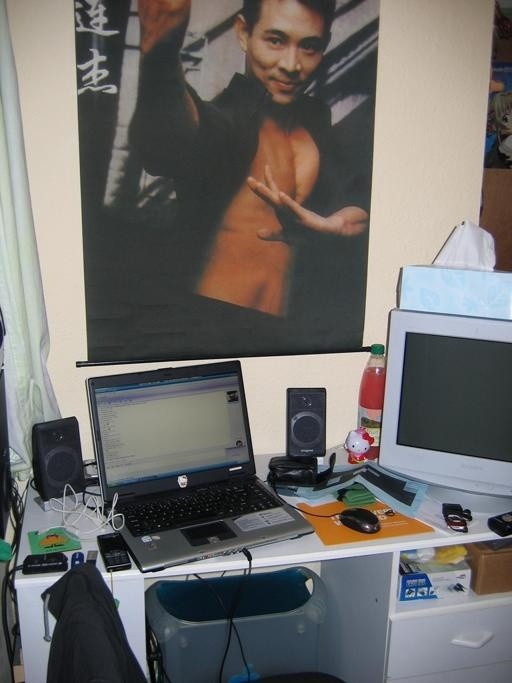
[14,445,512,682]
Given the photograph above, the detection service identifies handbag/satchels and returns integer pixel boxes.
[268,456,317,484]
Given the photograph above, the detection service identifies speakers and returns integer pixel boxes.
[31,416,85,501]
[287,388,326,457]
[85,361,315,573]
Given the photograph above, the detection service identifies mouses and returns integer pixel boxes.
[339,507,380,534]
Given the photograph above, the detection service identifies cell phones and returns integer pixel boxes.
[97,531,130,572]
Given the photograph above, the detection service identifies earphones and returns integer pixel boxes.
[461,510,471,516]
[461,515,472,521]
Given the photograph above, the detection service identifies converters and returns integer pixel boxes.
[21,552,68,574]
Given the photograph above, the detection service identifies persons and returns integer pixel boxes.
[128,0,372,359]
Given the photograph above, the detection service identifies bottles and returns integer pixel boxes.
[356,342,386,458]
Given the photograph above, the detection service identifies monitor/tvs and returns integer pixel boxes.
[378,308,512,518]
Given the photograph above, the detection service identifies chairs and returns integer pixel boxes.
[54,560,350,683]
